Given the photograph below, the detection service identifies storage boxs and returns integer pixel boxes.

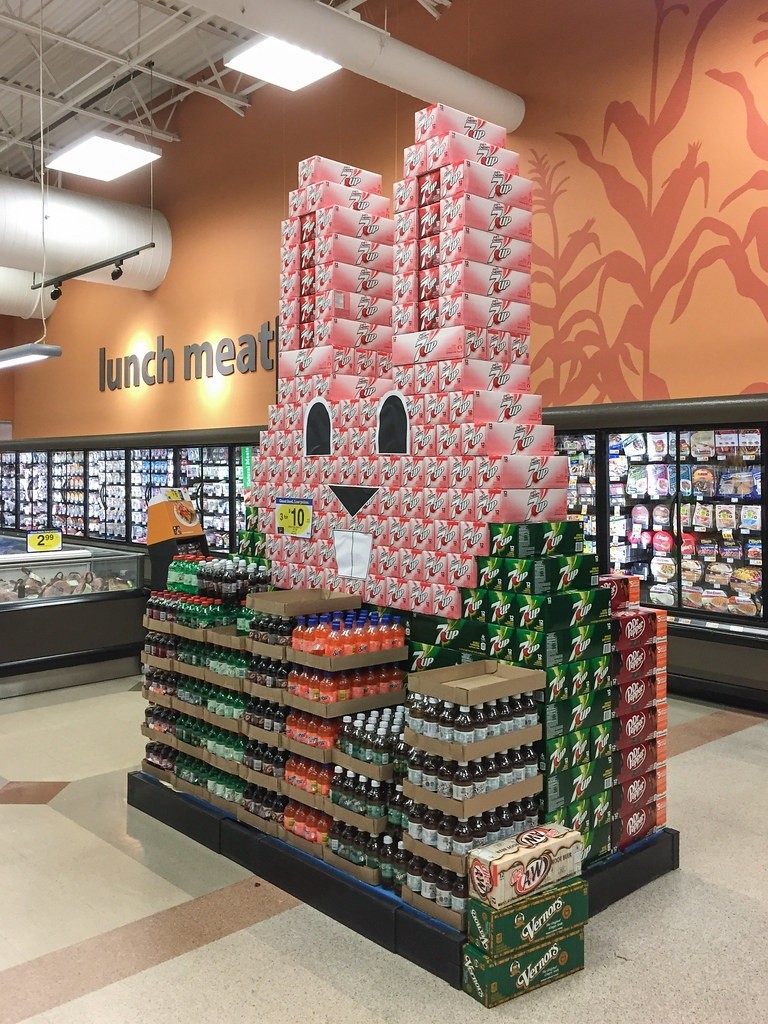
[139,99,666,1011]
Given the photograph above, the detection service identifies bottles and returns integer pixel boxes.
[141,552,538,913]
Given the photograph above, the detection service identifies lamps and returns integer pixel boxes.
[111,260,123,281]
[50,281,62,301]
[0,1,62,369]
[46,124,163,184]
[223,0,389,91]
